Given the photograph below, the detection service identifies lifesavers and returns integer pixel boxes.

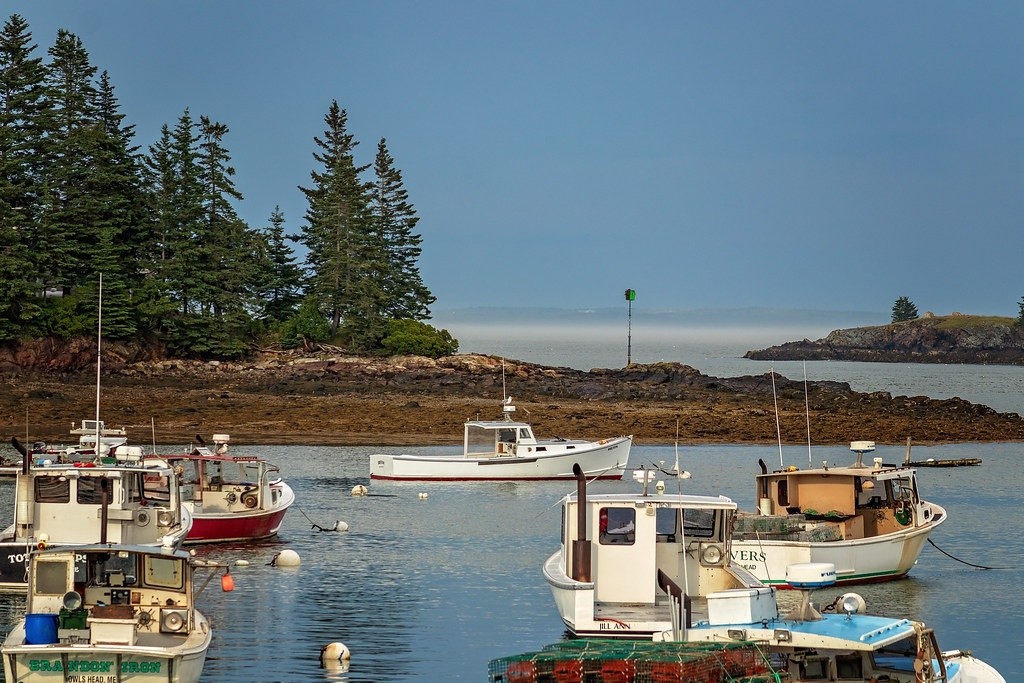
[90,442,96,448]
[73,462,97,468]
[600,510,608,532]
[81,442,86,446]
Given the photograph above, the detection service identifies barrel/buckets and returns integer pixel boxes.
[63,590,82,610]
[25,613,61,645]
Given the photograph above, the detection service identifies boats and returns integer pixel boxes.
[1,270,297,683]
[369,357,635,483]
[488,360,1005,683]
